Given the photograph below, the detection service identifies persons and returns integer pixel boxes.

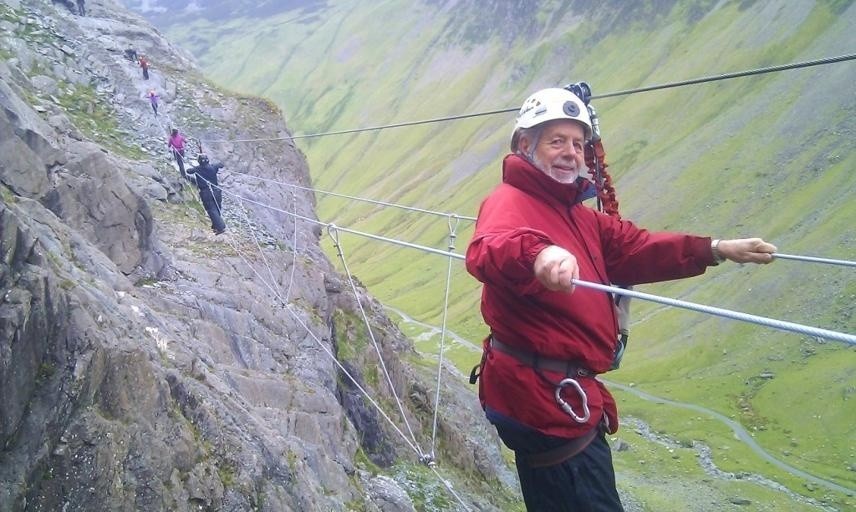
[169,130,188,179]
[146,91,160,116]
[52,0,86,15]
[463,88,778,512]
[123,43,137,61]
[187,154,226,232]
[139,56,148,79]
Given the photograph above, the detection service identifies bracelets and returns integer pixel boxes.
[709,239,725,264]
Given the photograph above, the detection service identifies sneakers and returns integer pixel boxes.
[213,227,226,235]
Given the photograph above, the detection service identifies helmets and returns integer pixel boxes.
[198,154,209,164]
[510,88,593,153]
